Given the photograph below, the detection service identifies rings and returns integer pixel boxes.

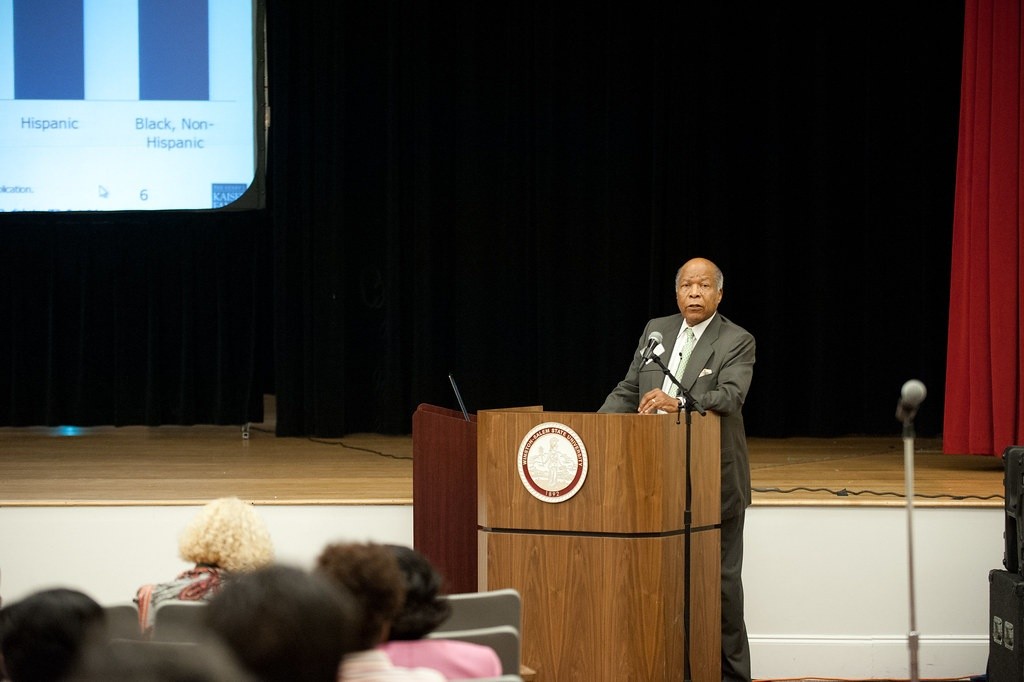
[652,399,655,402]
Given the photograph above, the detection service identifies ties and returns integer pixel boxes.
[656,328,695,414]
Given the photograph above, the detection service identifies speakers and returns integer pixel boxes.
[985,446,1024,682]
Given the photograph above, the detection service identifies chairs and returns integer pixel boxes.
[427,588,536,682]
[104,587,207,658]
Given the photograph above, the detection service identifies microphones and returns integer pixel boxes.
[638,331,663,373]
[896,378,929,421]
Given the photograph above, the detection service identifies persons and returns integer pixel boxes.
[148,498,271,626]
[317,544,444,682]
[377,545,502,679]
[598,257,755,682]
[80,621,241,681]
[210,563,348,682]
[0,589,107,682]
